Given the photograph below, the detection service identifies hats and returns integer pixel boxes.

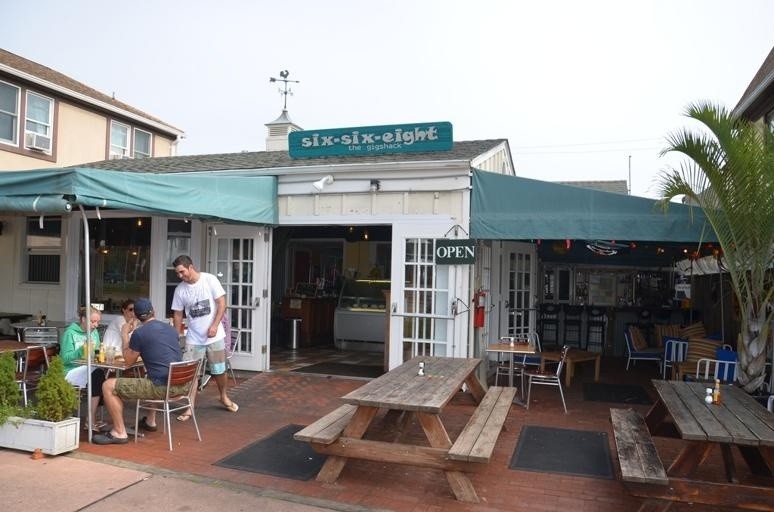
[133,298,153,319]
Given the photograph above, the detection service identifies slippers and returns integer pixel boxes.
[92,431,130,445]
[219,398,240,413]
[176,409,191,422]
[139,414,159,431]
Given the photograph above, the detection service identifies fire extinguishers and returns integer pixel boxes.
[474,286,489,328]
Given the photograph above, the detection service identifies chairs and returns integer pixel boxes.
[484,302,609,415]
[622,319,740,383]
[0,312,243,455]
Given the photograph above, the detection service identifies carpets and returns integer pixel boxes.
[291,358,385,379]
[511,422,616,479]
[211,418,322,482]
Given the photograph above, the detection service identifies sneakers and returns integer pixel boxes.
[83,417,107,432]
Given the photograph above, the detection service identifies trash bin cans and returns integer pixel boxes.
[284,317,304,350]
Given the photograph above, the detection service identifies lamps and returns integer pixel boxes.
[313,173,334,192]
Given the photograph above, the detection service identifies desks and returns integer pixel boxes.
[315,355,499,505]
[646,377,774,512]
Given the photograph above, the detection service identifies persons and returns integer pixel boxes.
[169,254,239,423]
[197,312,232,390]
[91,298,183,445]
[58,306,107,435]
[102,298,143,351]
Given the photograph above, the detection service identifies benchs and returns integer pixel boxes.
[435,384,518,472]
[607,405,672,501]
[294,403,362,456]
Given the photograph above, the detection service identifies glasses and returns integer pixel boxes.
[127,308,133,312]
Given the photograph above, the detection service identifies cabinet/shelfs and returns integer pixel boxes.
[332,276,390,353]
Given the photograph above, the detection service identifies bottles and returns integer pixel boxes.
[509,337,514,347]
[35,309,45,326]
[703,386,713,404]
[81,340,114,363]
[713,378,721,406]
[416,361,424,376]
